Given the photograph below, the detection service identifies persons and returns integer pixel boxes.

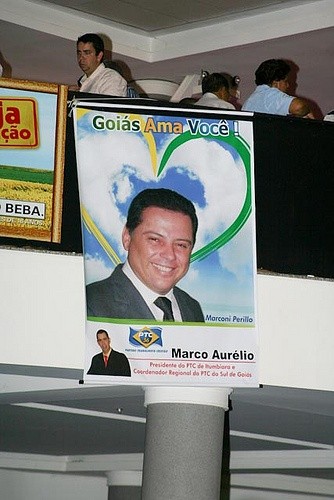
[76,33,139,97]
[193,72,236,110]
[87,329,131,377]
[241,59,315,119]
[84,188,206,321]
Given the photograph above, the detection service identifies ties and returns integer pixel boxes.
[105,356,108,366]
[153,297,175,322]
